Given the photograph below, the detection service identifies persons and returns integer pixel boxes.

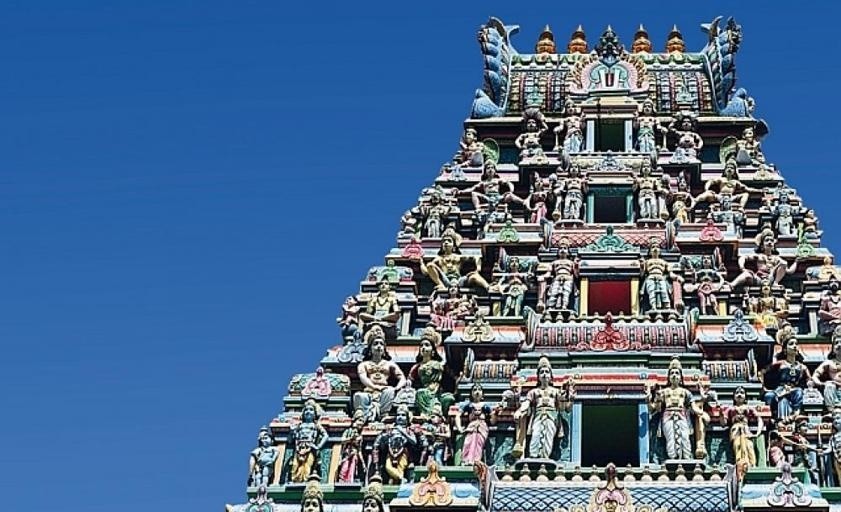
[248,99,841,512]
